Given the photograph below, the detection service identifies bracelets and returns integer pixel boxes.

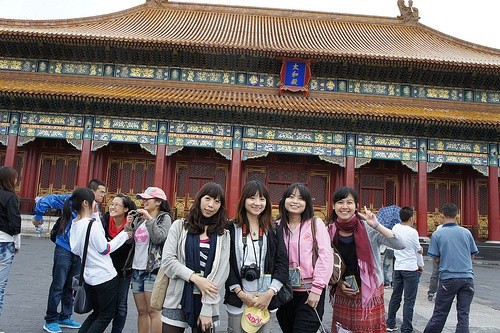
[243,294,247,303]
[374,223,379,229]
[236,290,241,295]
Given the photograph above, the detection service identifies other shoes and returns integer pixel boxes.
[384,284,389,289]
[393,282,394,288]
[428,295,433,300]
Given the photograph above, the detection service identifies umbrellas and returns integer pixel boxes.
[376,205,402,229]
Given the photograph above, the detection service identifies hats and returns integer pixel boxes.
[136,187,166,200]
[241,297,270,333]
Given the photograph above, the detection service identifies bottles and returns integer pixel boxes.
[36,224,41,238]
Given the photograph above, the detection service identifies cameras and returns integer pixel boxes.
[130,210,142,218]
[240,263,260,281]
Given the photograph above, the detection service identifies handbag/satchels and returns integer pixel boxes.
[72,275,93,314]
[49,214,63,243]
[268,224,293,310]
[311,217,346,285]
[150,218,187,311]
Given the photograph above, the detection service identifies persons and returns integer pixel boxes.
[162,182,230,333]
[0,166,22,314]
[325,186,406,333]
[222,181,289,333]
[59,187,131,333]
[276,182,334,333]
[125,187,172,333]
[386,206,424,333]
[428,224,443,300]
[381,245,396,289]
[32,180,106,333]
[98,193,137,333]
[422,204,479,333]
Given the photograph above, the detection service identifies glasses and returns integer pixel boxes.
[110,202,125,207]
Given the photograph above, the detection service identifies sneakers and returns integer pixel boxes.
[58,316,81,328]
[386,327,398,331]
[43,319,62,333]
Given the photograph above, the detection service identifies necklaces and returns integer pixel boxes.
[253,232,256,236]
